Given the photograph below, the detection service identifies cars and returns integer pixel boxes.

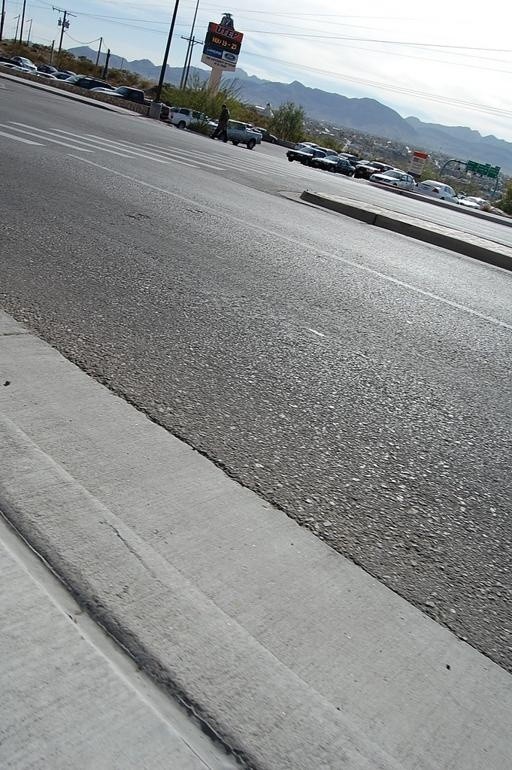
[285,140,512,218]
[0,51,171,119]
[167,104,280,149]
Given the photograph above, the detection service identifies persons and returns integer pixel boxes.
[209,104,230,143]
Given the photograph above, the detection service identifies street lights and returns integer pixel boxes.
[55,16,71,67]
